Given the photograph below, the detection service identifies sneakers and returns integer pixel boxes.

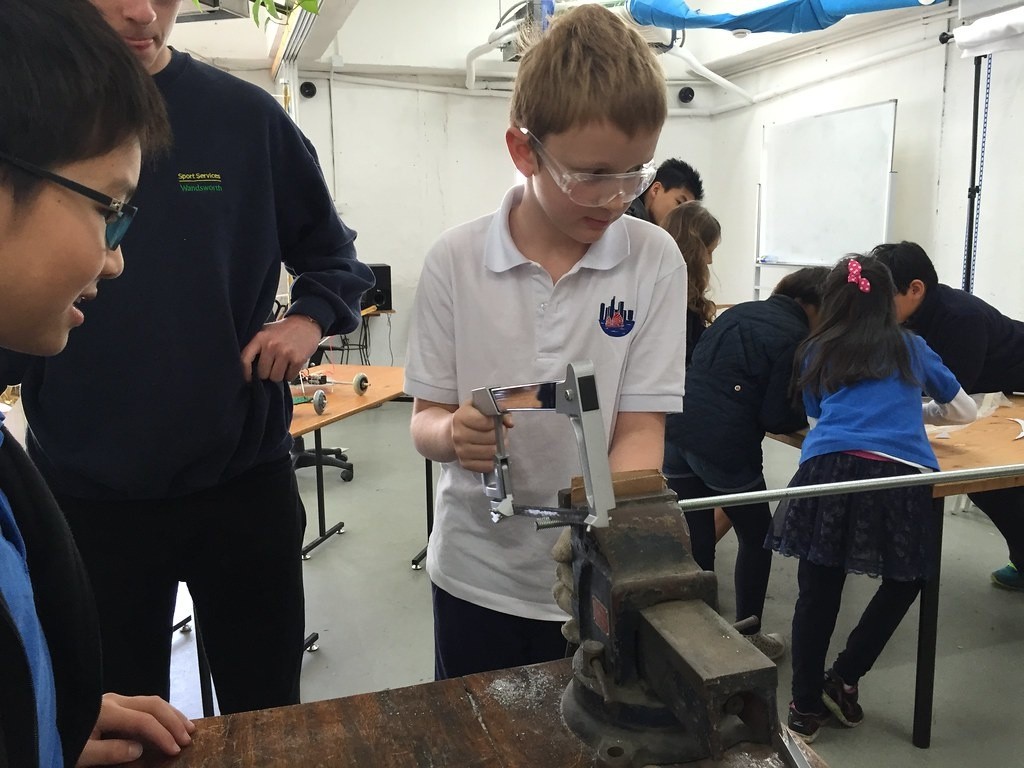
[990,562,1024,591]
[821,668,864,727]
[788,701,832,743]
[742,632,786,659]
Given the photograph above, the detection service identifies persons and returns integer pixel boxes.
[663,266,831,660]
[403,2,687,681]
[625,157,703,226]
[0,0,194,768]
[763,255,978,744]
[660,200,734,543]
[872,241,1024,590]
[0,0,377,716]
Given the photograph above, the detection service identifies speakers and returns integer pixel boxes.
[360,264,392,310]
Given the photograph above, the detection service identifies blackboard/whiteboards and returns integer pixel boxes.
[754,97,897,268]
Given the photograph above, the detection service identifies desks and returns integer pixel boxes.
[103,655,832,768]
[764,386,1024,749]
[283,364,408,561]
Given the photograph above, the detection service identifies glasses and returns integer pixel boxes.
[520,127,657,208]
[0,151,139,251]
